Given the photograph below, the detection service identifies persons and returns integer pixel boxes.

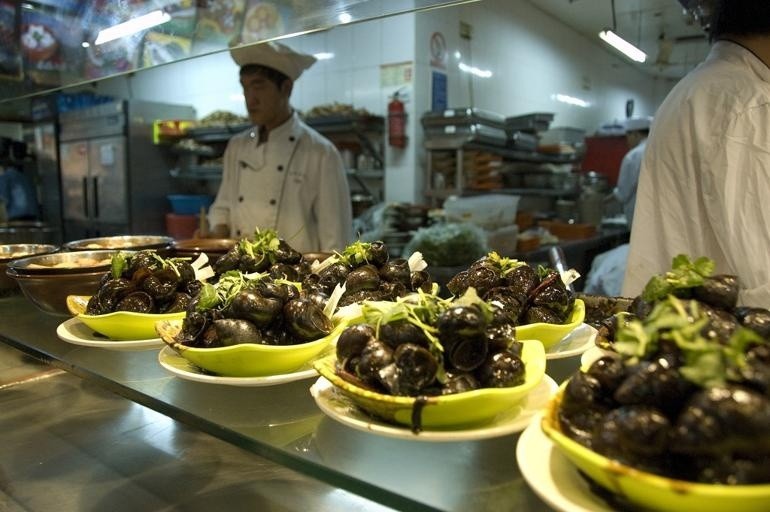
[193,37,354,254]
[616,0,770,312]
[602,118,651,232]
[583,240,630,298]
[1,158,37,230]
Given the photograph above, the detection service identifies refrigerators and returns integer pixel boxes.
[55,99,198,238]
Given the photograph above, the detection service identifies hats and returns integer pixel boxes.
[625,118,651,131]
[229,41,317,81]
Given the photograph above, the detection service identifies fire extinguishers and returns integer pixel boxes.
[388,84,410,146]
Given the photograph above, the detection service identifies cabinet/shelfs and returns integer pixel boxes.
[167,116,385,238]
[424,140,582,224]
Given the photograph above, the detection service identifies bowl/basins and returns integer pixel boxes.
[503,295,588,348]
[63,292,346,368]
[539,375,770,512]
[165,192,213,216]
[0,233,175,319]
[309,337,548,430]
[506,167,612,192]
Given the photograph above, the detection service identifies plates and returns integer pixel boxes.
[537,319,598,363]
[54,314,319,391]
[308,372,560,448]
[514,417,594,512]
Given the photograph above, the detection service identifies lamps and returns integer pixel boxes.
[599,0,648,64]
